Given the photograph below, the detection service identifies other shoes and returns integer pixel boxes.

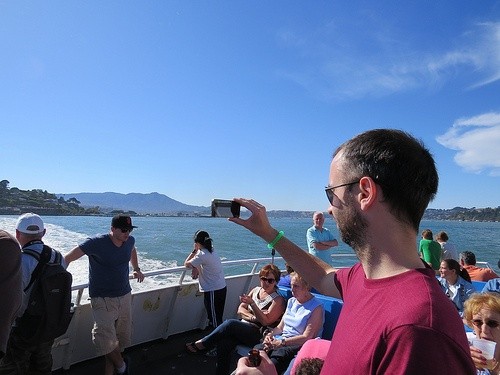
[116,360,128,375]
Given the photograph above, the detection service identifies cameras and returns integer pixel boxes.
[211,199,240,218]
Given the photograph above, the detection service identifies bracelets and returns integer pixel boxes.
[133,268,140,272]
[268,231,283,248]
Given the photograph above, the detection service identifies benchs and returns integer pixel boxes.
[237,285,343,359]
[436,276,487,332]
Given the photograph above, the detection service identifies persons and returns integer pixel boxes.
[185,267,285,375]
[419,230,441,270]
[6,212,67,375]
[459,252,497,282]
[276,263,294,288]
[463,292,500,375]
[0,231,21,354]
[437,259,476,325]
[185,231,227,327]
[481,259,500,293]
[65,213,145,375]
[307,211,338,266]
[435,232,458,262]
[228,128,479,375]
[253,272,325,375]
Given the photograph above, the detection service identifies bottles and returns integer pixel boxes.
[247,349,261,367]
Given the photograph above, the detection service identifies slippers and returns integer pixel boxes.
[184,341,206,356]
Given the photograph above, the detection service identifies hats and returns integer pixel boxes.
[15,212,44,234]
[111,213,138,228]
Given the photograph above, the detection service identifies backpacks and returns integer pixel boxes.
[14,244,72,342]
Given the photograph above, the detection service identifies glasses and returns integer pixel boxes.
[325,178,381,205]
[122,228,133,233]
[471,318,500,327]
[261,277,275,284]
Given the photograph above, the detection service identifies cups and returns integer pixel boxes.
[473,339,497,369]
